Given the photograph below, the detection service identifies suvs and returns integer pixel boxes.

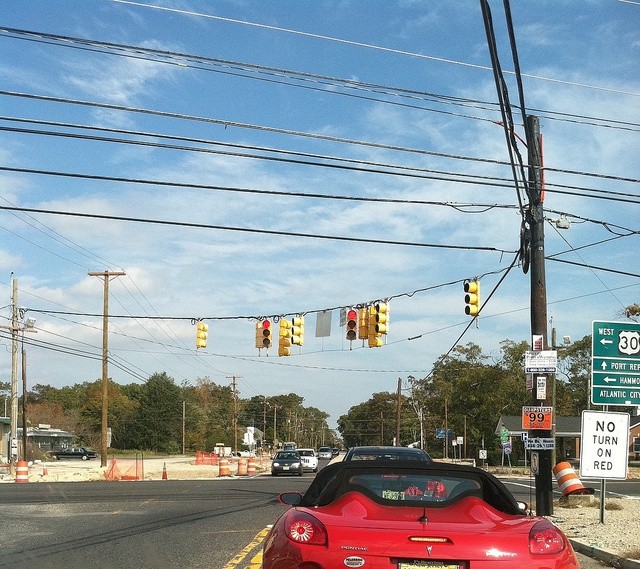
[295,448,319,472]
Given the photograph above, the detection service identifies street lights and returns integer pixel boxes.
[551,327,572,470]
[21,316,37,460]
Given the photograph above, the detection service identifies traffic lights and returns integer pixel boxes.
[291,316,304,346]
[278,320,292,356]
[262,320,273,348]
[464,281,480,317]
[346,310,357,341]
[196,322,209,349]
[374,301,390,335]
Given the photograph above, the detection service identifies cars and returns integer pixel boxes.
[47,446,98,461]
[332,448,339,455]
[342,445,449,503]
[317,447,333,460]
[270,450,303,475]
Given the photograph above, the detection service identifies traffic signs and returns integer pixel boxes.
[591,386,640,406]
[592,357,640,374]
[591,371,640,387]
[592,320,640,359]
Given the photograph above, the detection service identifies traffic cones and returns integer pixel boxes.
[162,462,169,480]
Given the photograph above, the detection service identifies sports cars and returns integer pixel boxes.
[262,460,581,569]
